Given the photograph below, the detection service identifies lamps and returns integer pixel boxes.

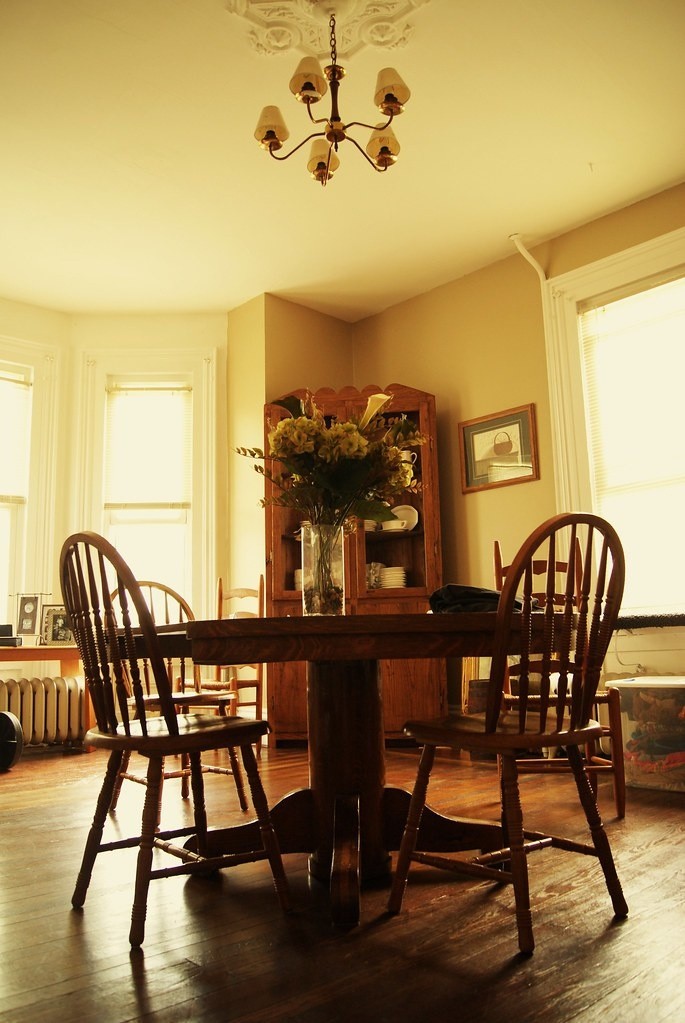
[254,13,410,187]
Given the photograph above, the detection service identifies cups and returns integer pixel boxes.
[381,520,407,530]
[400,450,417,464]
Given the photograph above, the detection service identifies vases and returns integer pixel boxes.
[301,525,346,616]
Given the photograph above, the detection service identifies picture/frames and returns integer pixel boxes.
[457,402,540,495]
[48,610,76,646]
[39,605,65,645]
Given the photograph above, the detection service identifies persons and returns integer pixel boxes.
[52,614,70,641]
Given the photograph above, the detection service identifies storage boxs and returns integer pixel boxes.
[604,675,685,792]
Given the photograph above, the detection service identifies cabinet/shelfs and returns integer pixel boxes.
[263,382,449,750]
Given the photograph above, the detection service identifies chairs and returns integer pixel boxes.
[60,531,292,947]
[102,581,249,812]
[177,575,263,757]
[382,512,630,955]
[493,539,627,819]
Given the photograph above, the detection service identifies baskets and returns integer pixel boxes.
[494,432,513,455]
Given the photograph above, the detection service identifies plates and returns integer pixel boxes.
[379,567,407,589]
[389,505,419,531]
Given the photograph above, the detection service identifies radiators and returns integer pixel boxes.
[0,677,82,743]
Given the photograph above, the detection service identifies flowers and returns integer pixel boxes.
[230,391,433,522]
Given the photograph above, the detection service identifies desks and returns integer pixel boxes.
[182,612,580,895]
[0,645,82,677]
